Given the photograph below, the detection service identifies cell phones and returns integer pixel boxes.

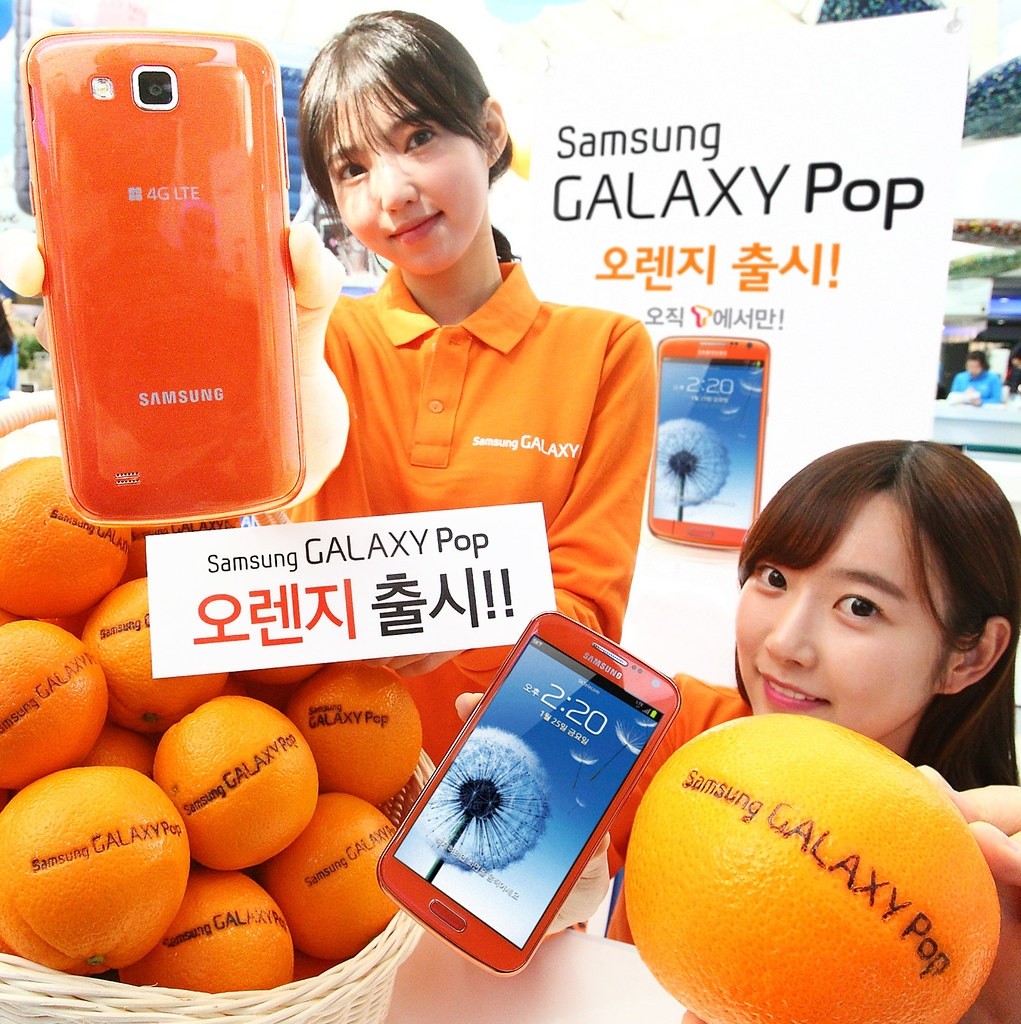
[376,609,682,976]
[24,24,306,528]
[649,338,771,551]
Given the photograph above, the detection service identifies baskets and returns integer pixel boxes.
[0,750,441,1024]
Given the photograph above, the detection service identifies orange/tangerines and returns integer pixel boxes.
[626,712,1001,1024]
[0,457,423,995]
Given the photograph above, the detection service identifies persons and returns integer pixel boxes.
[0,295,21,401]
[946,350,1003,406]
[453,439,1021,1024]
[0,7,658,932]
[1004,349,1021,394]
